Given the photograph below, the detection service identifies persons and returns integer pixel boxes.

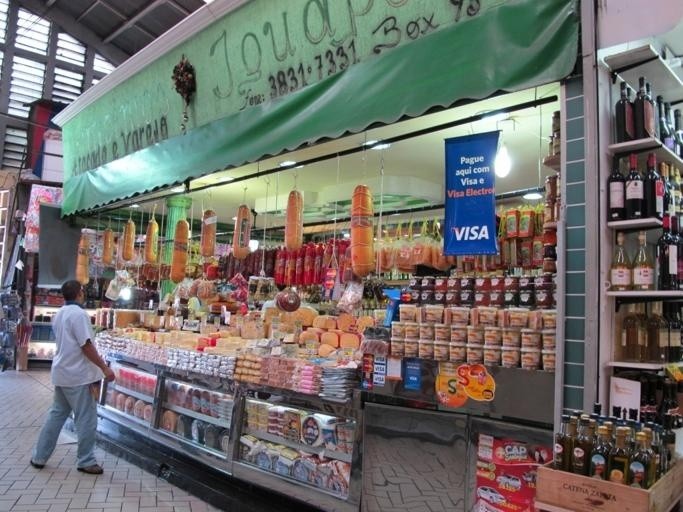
[29,280,116,475]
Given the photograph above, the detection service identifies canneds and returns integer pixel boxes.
[400,173,561,310]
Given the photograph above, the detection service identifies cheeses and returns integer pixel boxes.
[241,304,374,357]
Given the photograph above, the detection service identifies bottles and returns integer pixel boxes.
[619,300,682,362]
[620,369,682,429]
[546,109,561,157]
[615,77,682,159]
[609,213,683,291]
[605,152,682,221]
[552,402,675,490]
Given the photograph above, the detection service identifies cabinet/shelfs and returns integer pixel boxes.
[600,50,681,428]
[91,345,361,511]
[351,344,553,510]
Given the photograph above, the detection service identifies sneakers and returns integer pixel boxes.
[78,465,103,474]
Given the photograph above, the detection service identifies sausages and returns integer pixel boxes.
[233,204,252,260]
[122,221,135,260]
[145,218,158,263]
[171,220,190,283]
[103,229,113,265]
[351,186,375,282]
[75,236,90,284]
[273,239,354,284]
[200,210,217,256]
[284,190,304,249]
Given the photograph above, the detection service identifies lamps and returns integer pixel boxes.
[248,208,261,253]
[493,119,518,177]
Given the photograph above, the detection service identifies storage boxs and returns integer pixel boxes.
[535,452,682,512]
